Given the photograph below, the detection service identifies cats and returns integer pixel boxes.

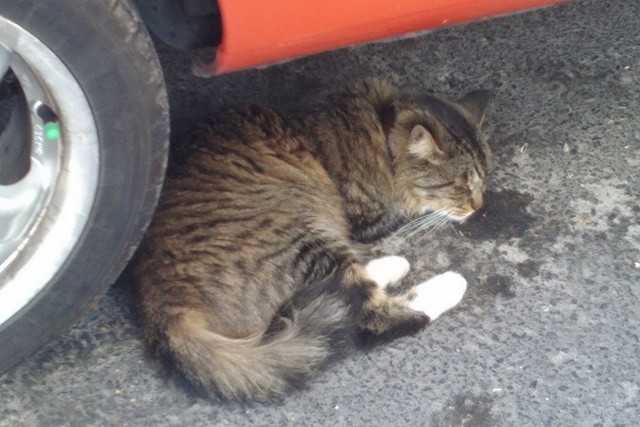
[130,80,493,404]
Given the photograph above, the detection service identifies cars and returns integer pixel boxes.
[0,0,565,376]
